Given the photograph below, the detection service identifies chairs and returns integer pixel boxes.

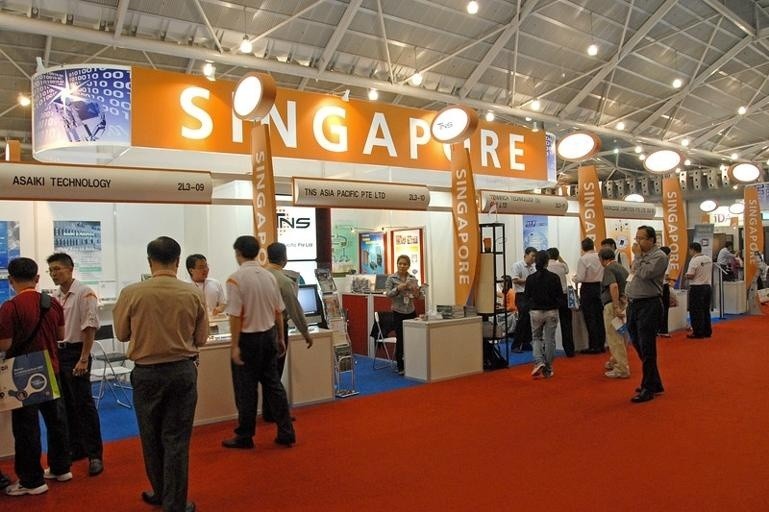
[88,324,133,410]
[372,311,397,369]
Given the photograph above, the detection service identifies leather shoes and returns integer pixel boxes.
[186,500,195,512]
[635,385,664,394]
[222,435,254,448]
[631,383,655,402]
[89,458,103,474]
[265,413,296,422]
[511,347,524,352]
[142,490,162,504]
[688,333,704,339]
[275,437,295,447]
[580,346,601,353]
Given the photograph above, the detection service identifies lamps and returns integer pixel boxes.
[233,70,276,267]
[412,45,423,84]
[557,130,605,259]
[333,89,350,102]
[382,226,408,232]
[727,161,763,288]
[672,50,681,89]
[430,104,478,308]
[699,199,717,224]
[587,11,598,56]
[729,203,745,226]
[532,77,540,111]
[35,56,46,74]
[736,76,746,116]
[643,147,686,286]
[532,122,538,132]
[241,8,253,52]
[351,226,375,232]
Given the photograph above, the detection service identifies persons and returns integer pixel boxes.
[601,238,631,274]
[571,238,606,354]
[185,254,227,334]
[511,248,536,354]
[598,247,630,378]
[685,242,712,339]
[1,257,73,496]
[261,242,313,423]
[221,236,297,448]
[525,250,563,377]
[488,275,518,343]
[113,236,210,511]
[657,247,671,338]
[625,226,669,402]
[546,248,575,358]
[384,255,419,375]
[46,253,105,474]
[717,241,740,281]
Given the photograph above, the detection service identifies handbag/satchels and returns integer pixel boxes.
[0,298,60,413]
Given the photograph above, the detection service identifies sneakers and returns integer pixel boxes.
[44,467,73,482]
[605,365,630,378]
[604,361,615,369]
[542,371,554,377]
[5,479,48,495]
[531,361,545,377]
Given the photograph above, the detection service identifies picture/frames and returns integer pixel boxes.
[389,228,424,286]
[358,233,386,275]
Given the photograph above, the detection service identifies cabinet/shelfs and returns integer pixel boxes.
[723,281,747,315]
[403,316,483,383]
[317,277,361,398]
[555,308,587,351]
[340,293,428,363]
[289,330,334,406]
[479,224,509,370]
[657,288,687,337]
[190,340,237,425]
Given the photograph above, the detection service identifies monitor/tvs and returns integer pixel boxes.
[287,282,323,328]
